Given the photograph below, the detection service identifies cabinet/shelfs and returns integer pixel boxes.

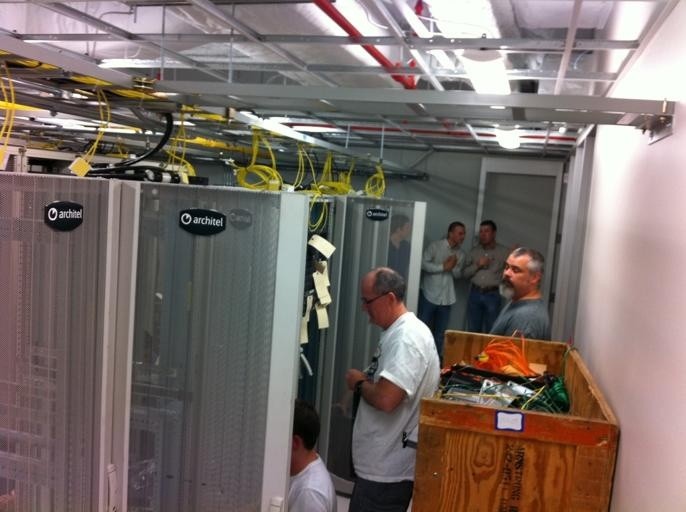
[411,329,620,512]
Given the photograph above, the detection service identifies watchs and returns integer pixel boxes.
[354,379,369,394]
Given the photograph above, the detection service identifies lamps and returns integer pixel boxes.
[494,126,520,149]
[459,47,512,97]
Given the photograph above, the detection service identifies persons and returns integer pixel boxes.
[489,246,551,342]
[387,214,411,282]
[345,266,441,512]
[287,398,338,512]
[417,221,467,353]
[462,219,511,334]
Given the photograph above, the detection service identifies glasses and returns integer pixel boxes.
[361,291,392,304]
